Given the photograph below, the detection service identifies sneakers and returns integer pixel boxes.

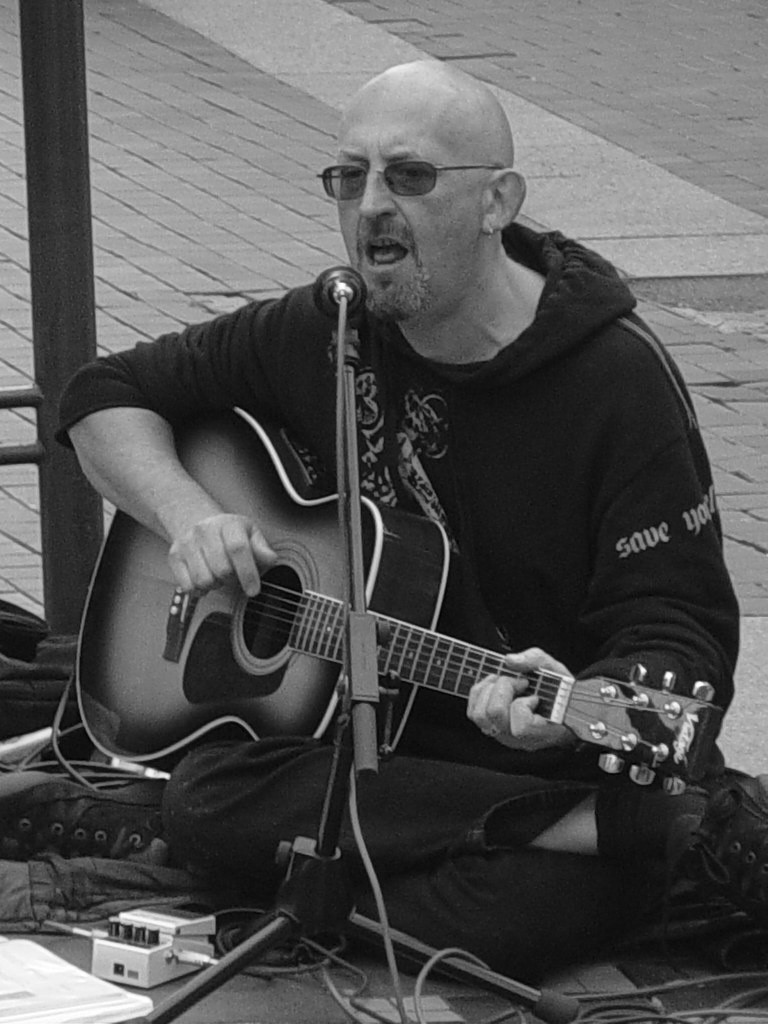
[671,766,768,927]
[0,770,168,861]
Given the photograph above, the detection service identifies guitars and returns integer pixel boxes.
[71,402,729,800]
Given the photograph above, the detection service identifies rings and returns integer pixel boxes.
[481,724,499,737]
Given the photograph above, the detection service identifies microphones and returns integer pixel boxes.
[313,266,368,320]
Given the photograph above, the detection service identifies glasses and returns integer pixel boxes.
[317,160,505,201]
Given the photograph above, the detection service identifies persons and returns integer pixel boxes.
[54,61,768,993]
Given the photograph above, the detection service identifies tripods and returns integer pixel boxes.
[116,306,582,1024]
